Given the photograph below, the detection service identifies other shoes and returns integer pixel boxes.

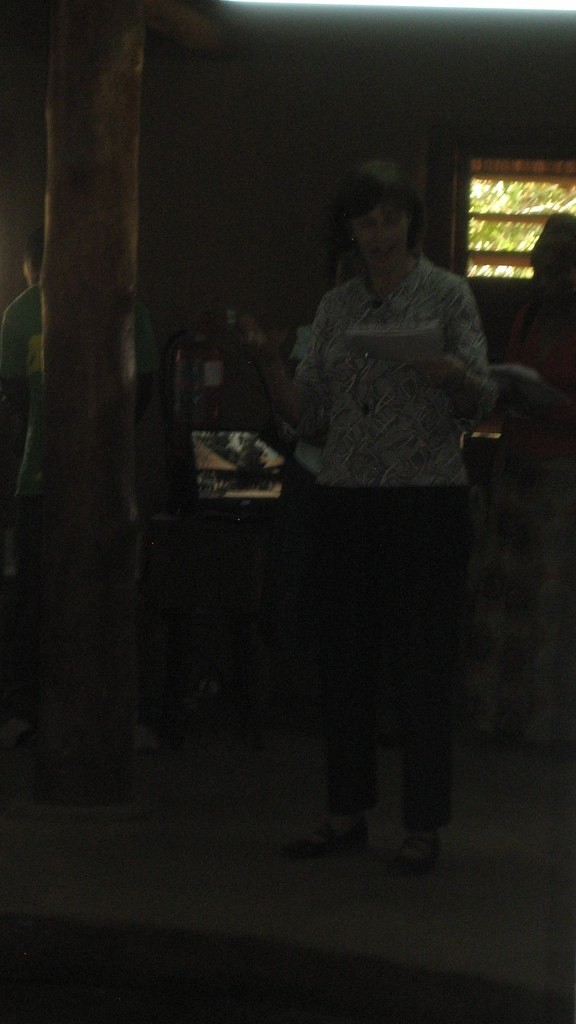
[287,820,373,864]
[393,833,446,879]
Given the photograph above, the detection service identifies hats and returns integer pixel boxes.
[530,213,576,264]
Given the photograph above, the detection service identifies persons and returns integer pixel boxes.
[2,227,157,757]
[241,162,493,880]
[497,225,576,758]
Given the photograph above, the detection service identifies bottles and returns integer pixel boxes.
[169,343,192,426]
[201,348,225,421]
